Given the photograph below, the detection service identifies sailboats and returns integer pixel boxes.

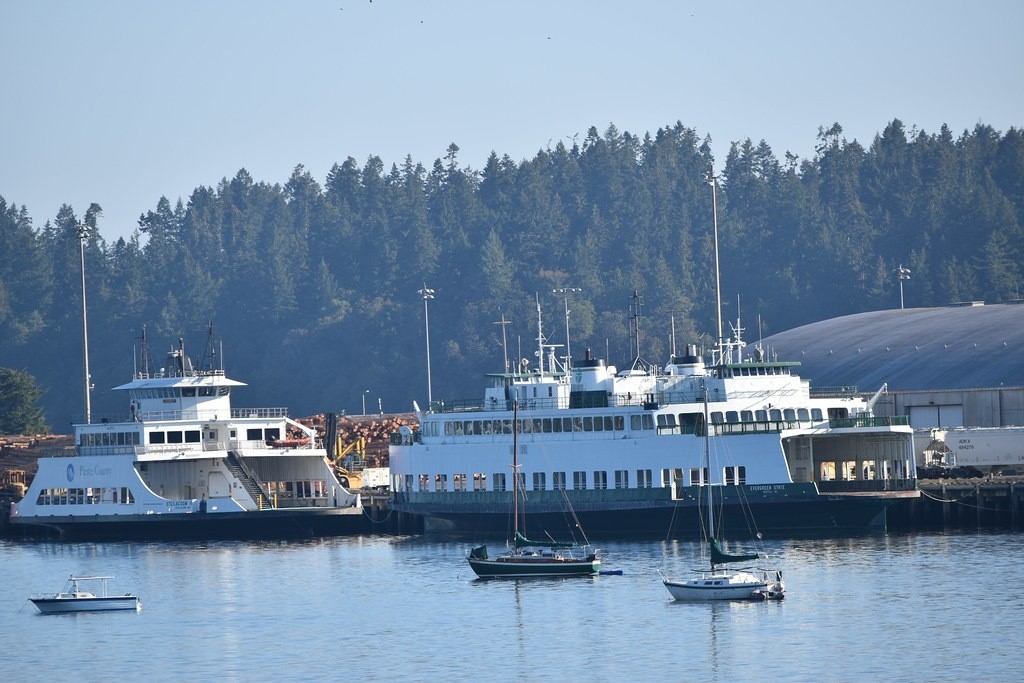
[464,387,601,578]
[658,387,788,601]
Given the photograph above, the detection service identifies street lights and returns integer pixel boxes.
[76,219,95,425]
[419,283,434,413]
[898,264,911,309]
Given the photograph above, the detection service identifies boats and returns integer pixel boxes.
[389,172,921,541]
[28,574,141,614]
[7,322,364,534]
